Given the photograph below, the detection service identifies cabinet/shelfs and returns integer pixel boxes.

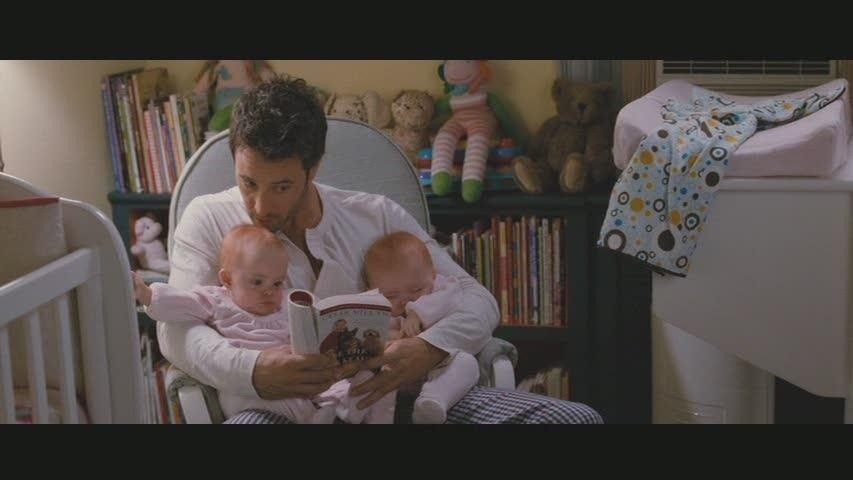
[107,177,622,423]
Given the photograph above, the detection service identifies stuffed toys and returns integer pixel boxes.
[194,61,278,142]
[431,61,508,202]
[380,89,436,166]
[313,87,393,131]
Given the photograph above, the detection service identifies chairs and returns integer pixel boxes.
[165,114,519,425]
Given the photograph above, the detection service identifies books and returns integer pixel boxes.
[138,330,181,424]
[101,67,210,197]
[439,215,571,328]
[513,362,572,400]
[286,285,394,382]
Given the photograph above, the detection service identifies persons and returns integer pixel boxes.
[129,224,376,424]
[319,316,358,364]
[154,72,605,425]
[360,230,483,426]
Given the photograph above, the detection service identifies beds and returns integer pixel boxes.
[0,171,151,424]
